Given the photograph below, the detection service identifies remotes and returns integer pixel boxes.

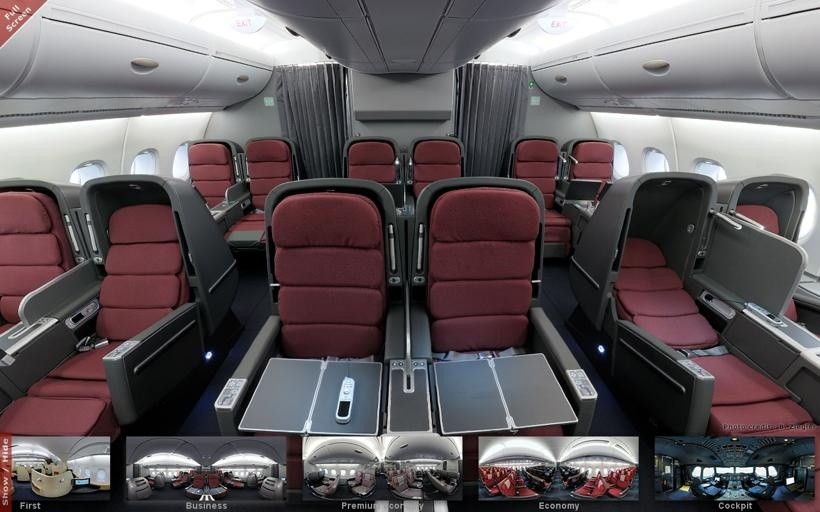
[336,377,355,423]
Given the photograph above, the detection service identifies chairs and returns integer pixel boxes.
[1,179,83,348]
[212,136,299,256]
[215,179,408,437]
[348,472,362,486]
[568,172,820,511]
[11,459,74,498]
[313,474,340,495]
[170,471,183,486]
[385,469,460,499]
[561,138,617,260]
[173,474,189,489]
[224,472,245,489]
[716,176,820,330]
[479,466,637,500]
[404,136,466,215]
[352,474,375,496]
[341,136,404,216]
[184,474,231,502]
[505,135,572,257]
[148,473,165,489]
[408,177,599,436]
[690,474,776,501]
[1,174,239,447]
[185,139,243,215]
